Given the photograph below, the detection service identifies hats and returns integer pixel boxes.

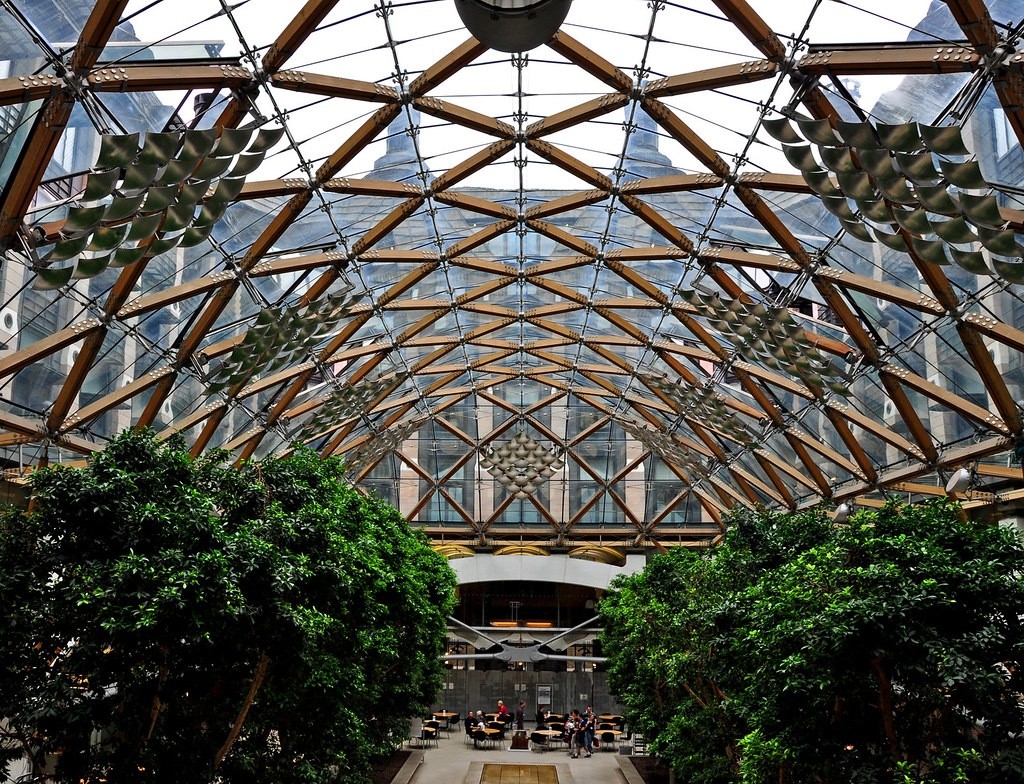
[498,700,503,704]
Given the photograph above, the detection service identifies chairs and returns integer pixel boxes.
[409,712,632,754]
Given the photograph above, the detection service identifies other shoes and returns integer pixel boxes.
[589,749,594,754]
[584,754,591,758]
[571,754,578,758]
[573,752,581,756]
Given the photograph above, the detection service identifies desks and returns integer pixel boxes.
[548,722,565,726]
[532,730,562,752]
[599,723,617,726]
[485,714,500,720]
[544,715,563,719]
[472,728,500,747]
[598,715,623,722]
[417,727,436,748]
[593,729,623,749]
[488,721,506,725]
[432,713,459,729]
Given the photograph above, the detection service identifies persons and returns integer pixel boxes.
[497,700,509,739]
[464,711,483,748]
[516,700,527,736]
[476,710,489,728]
[561,703,595,759]
[535,705,551,730]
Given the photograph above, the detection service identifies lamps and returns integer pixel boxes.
[831,504,856,525]
[945,468,974,502]
